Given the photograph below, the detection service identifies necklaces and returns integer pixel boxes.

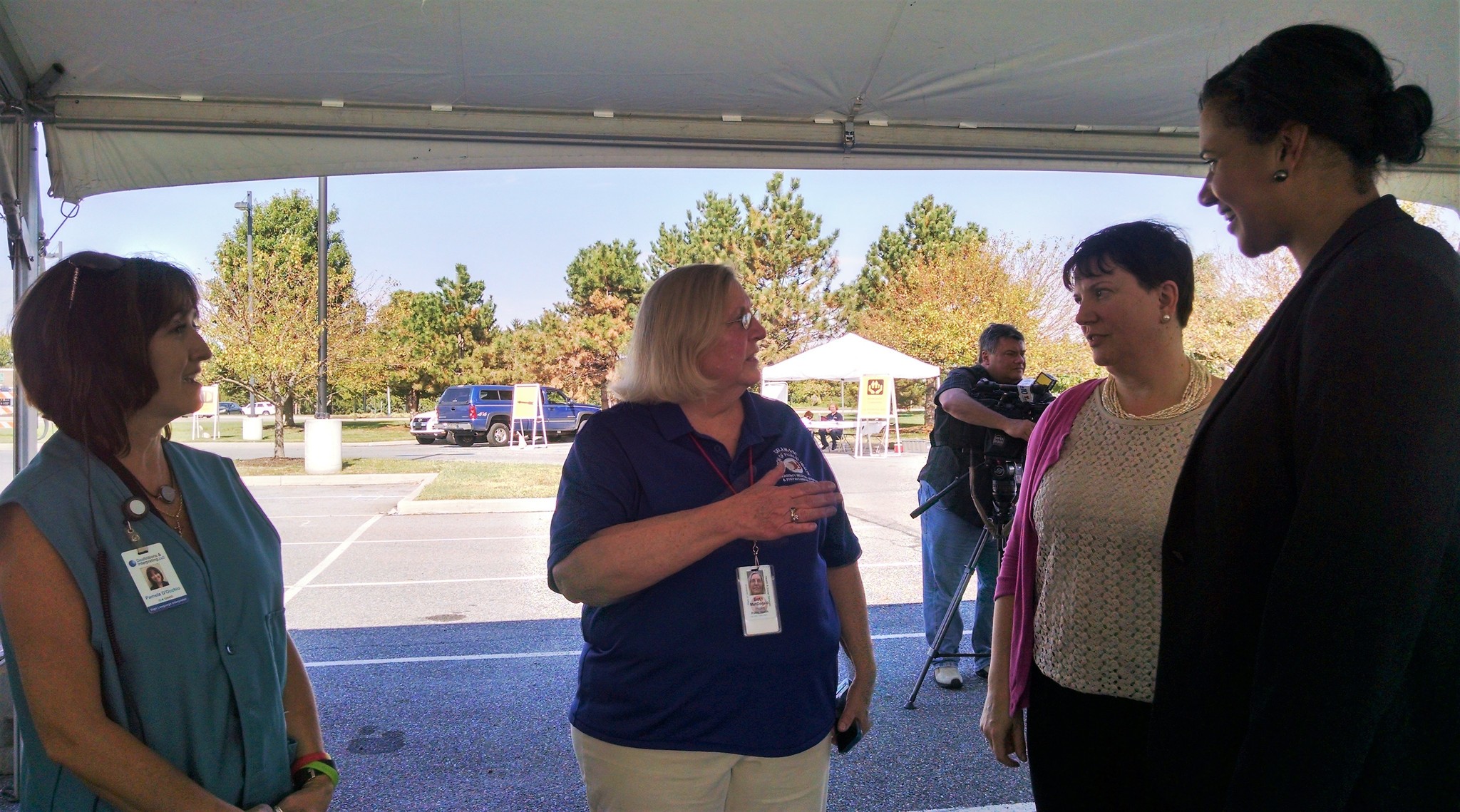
[1101,357,1212,419]
[137,455,185,536]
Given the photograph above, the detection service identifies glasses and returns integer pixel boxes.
[63,250,124,318]
[717,309,761,329]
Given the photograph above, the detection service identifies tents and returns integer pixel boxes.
[761,333,940,455]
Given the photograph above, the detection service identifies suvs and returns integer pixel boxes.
[241,401,276,416]
[410,405,458,445]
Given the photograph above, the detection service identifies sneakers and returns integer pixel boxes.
[975,664,991,681]
[934,666,962,686]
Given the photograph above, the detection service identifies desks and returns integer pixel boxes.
[806,421,887,455]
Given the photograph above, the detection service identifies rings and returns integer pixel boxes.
[274,806,283,812]
[789,507,798,524]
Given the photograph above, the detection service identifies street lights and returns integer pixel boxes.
[233,190,258,417]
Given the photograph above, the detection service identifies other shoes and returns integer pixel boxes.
[822,444,829,450]
[832,444,837,450]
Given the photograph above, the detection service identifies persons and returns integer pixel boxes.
[980,216,1228,812]
[146,567,170,590]
[916,323,1037,686]
[748,571,766,596]
[818,403,843,450]
[0,251,339,812]
[800,411,814,438]
[1147,18,1460,812]
[547,266,878,810]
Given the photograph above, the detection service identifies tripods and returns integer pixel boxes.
[902,476,1018,708]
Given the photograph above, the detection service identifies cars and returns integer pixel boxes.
[219,401,243,415]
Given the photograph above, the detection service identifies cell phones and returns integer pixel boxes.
[835,678,863,755]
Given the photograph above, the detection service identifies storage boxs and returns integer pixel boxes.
[894,444,903,453]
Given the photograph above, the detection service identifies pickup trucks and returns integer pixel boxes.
[436,385,602,447]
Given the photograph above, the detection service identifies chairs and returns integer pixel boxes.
[813,427,886,454]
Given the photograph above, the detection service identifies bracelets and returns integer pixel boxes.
[290,751,339,789]
